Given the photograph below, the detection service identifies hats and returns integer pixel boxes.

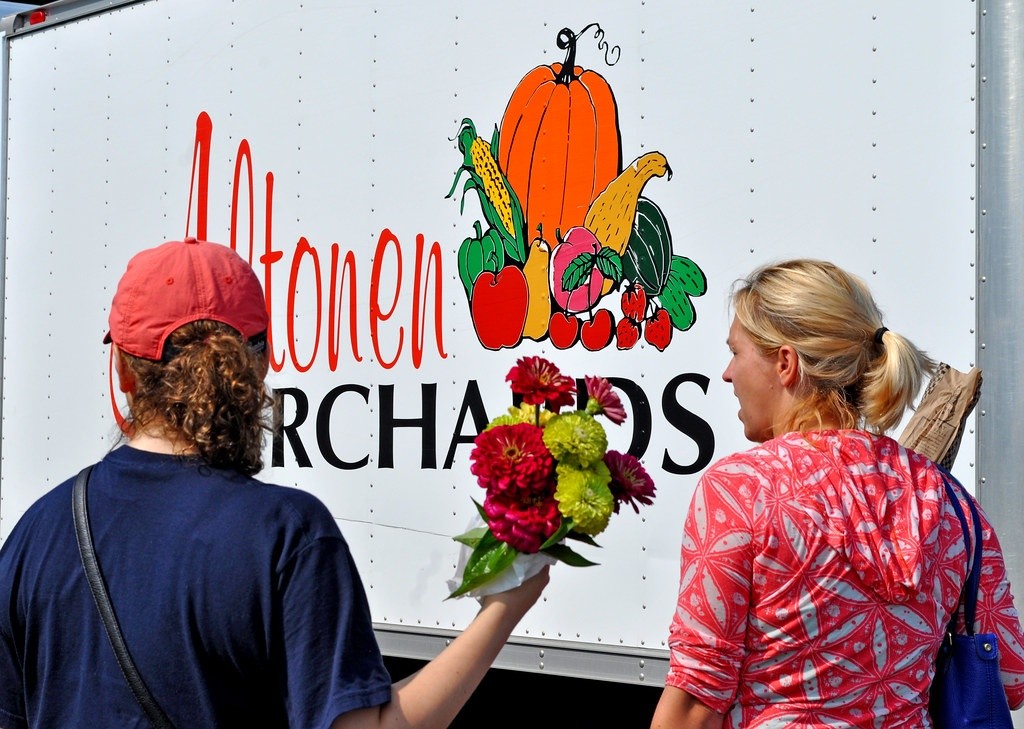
[103,238,269,360]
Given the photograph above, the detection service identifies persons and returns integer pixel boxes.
[642,257,1024,728]
[1,234,552,728]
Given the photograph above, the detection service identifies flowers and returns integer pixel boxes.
[441,355,657,603]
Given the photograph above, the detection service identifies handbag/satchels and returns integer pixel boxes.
[930,469,1014,729]
